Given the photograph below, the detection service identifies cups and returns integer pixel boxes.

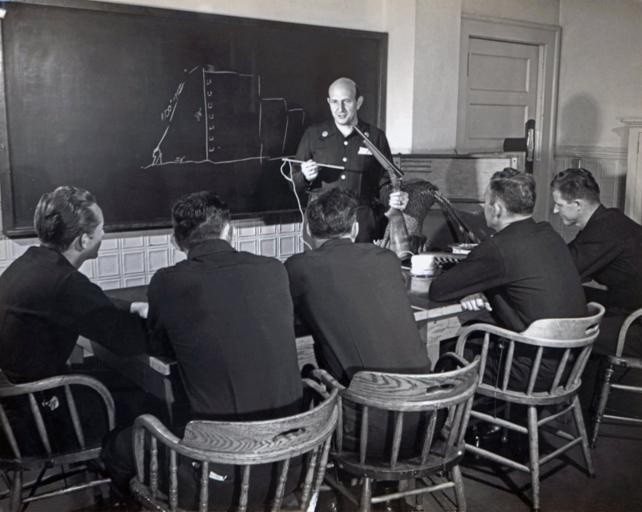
[411,255,435,275]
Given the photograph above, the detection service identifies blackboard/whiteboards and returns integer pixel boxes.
[2,0,388,238]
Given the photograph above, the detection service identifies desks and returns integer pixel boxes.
[66,250,468,402]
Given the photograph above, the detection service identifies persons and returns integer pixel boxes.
[428,166,590,465]
[95,191,307,512]
[1,184,152,458]
[542,165,642,443]
[281,192,433,512]
[286,78,410,246]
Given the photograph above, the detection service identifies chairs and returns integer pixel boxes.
[130,379,339,511]
[2,372,116,510]
[311,352,481,511]
[592,308,641,449]
[439,302,605,511]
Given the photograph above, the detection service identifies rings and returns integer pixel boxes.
[398,200,403,205]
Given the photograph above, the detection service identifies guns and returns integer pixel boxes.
[349,119,412,263]
